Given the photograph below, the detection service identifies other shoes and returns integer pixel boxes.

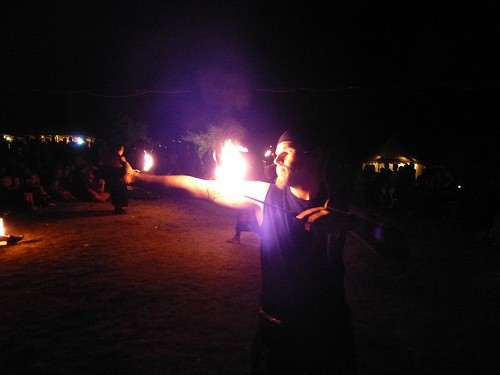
[114,208,126,213]
[227,236,240,243]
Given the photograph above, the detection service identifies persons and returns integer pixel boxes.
[1,134,195,222]
[223,204,263,245]
[354,157,450,216]
[120,127,400,374]
